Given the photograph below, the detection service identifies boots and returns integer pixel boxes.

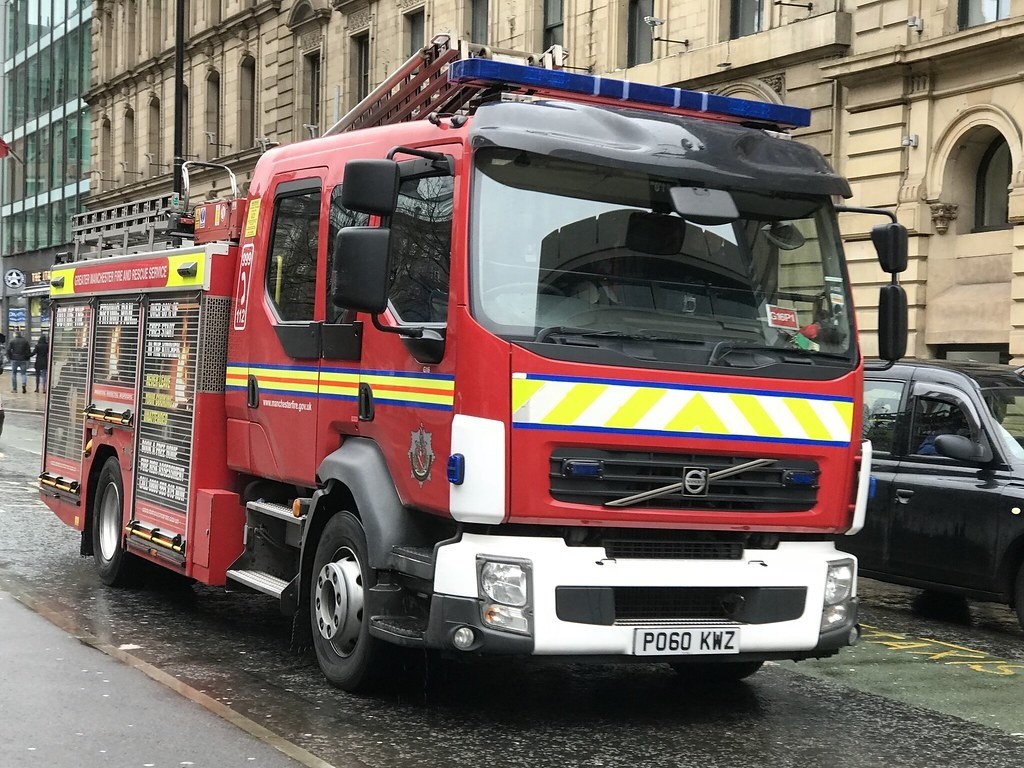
[43,383,46,393]
[34,382,39,392]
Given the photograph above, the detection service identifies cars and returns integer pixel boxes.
[829,355,1024,637]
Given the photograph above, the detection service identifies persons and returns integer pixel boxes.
[6,332,31,394]
[31,334,49,394]
[916,406,970,455]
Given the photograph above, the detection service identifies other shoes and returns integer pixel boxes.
[22,384,27,394]
[11,389,18,393]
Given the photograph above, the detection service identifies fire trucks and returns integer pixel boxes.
[39,31,909,702]
